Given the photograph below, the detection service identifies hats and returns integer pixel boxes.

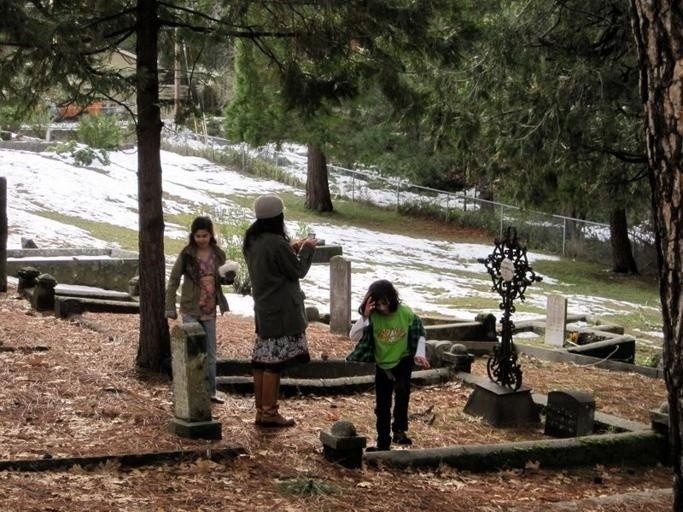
[191,216,213,233]
[255,194,283,219]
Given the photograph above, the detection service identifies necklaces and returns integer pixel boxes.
[199,256,200,259]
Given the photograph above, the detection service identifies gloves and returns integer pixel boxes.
[164,311,178,319]
[224,270,237,280]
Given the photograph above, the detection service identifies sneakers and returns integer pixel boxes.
[366,445,390,452]
[392,432,412,445]
[211,396,225,404]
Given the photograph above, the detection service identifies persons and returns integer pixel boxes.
[346,280,431,451]
[164,217,236,404]
[242,192,319,427]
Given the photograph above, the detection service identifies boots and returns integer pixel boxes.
[253,369,294,426]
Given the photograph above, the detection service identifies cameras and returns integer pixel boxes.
[307,234,316,239]
